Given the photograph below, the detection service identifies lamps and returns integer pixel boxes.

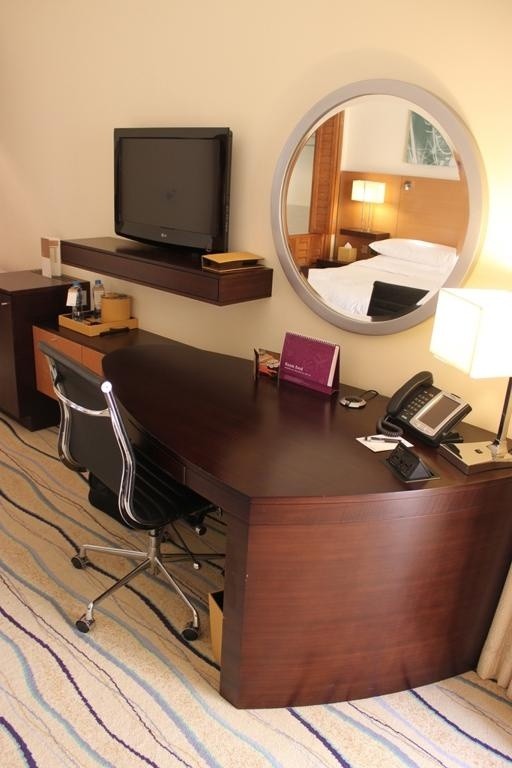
[351,179,386,230]
[428,285,511,477]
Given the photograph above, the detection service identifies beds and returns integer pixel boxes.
[306,254,458,315]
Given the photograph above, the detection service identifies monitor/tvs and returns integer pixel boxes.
[113,127,233,257]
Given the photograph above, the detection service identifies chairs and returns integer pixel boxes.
[367,279,429,315]
[36,339,226,642]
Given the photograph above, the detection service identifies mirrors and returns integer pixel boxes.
[270,76,487,337]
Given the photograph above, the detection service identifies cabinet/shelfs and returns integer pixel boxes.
[0,268,187,432]
[59,237,272,308]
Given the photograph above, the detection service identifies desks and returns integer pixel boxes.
[102,346,512,711]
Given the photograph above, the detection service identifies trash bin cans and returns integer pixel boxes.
[208,588,223,667]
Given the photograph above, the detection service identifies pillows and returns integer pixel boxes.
[368,238,456,268]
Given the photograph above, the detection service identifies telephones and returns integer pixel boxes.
[387,371,472,448]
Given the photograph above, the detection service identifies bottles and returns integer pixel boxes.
[70,282,84,321]
[91,280,106,317]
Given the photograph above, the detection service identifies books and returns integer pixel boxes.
[200,252,266,274]
